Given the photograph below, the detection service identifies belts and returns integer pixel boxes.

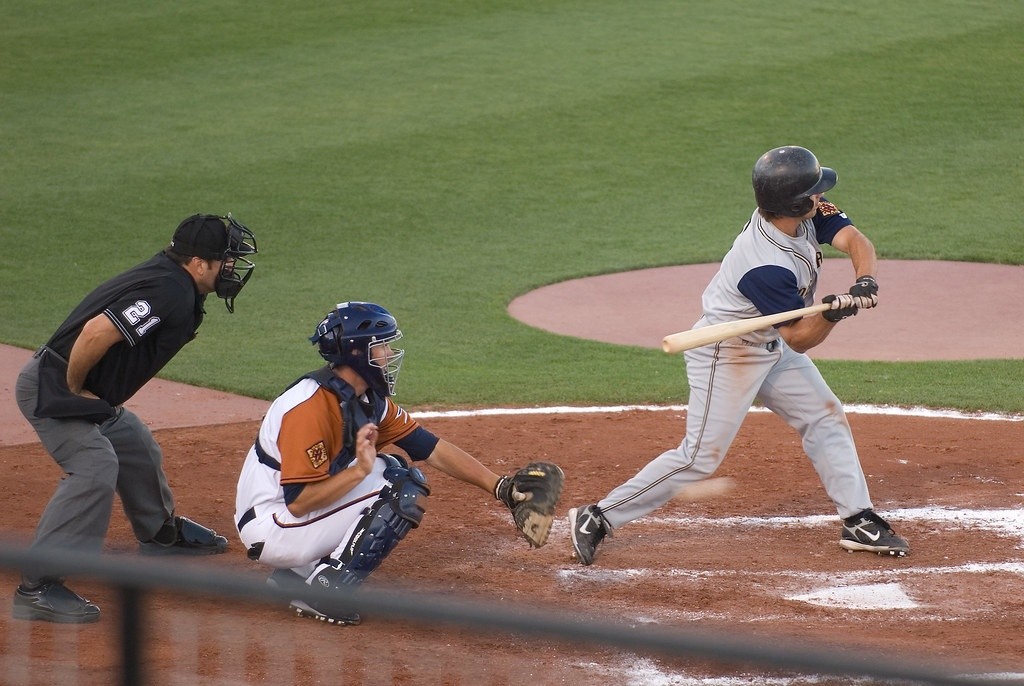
[766,340,777,352]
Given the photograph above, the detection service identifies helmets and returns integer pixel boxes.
[752,146,838,217]
[315,302,404,396]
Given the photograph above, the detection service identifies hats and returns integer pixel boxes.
[170,213,252,260]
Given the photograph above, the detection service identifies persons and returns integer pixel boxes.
[233,301,564,626]
[567,145,910,565]
[11,212,258,623]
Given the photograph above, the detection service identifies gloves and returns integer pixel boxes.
[821,293,858,322]
[849,275,879,309]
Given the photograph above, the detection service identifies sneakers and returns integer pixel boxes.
[266,567,308,593]
[289,586,361,626]
[569,504,613,566]
[839,510,909,556]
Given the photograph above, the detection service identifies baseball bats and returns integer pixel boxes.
[662,302,833,356]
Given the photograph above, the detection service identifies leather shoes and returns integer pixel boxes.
[12,581,101,624]
[138,516,228,555]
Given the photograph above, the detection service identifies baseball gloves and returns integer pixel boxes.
[495,461,566,549]
